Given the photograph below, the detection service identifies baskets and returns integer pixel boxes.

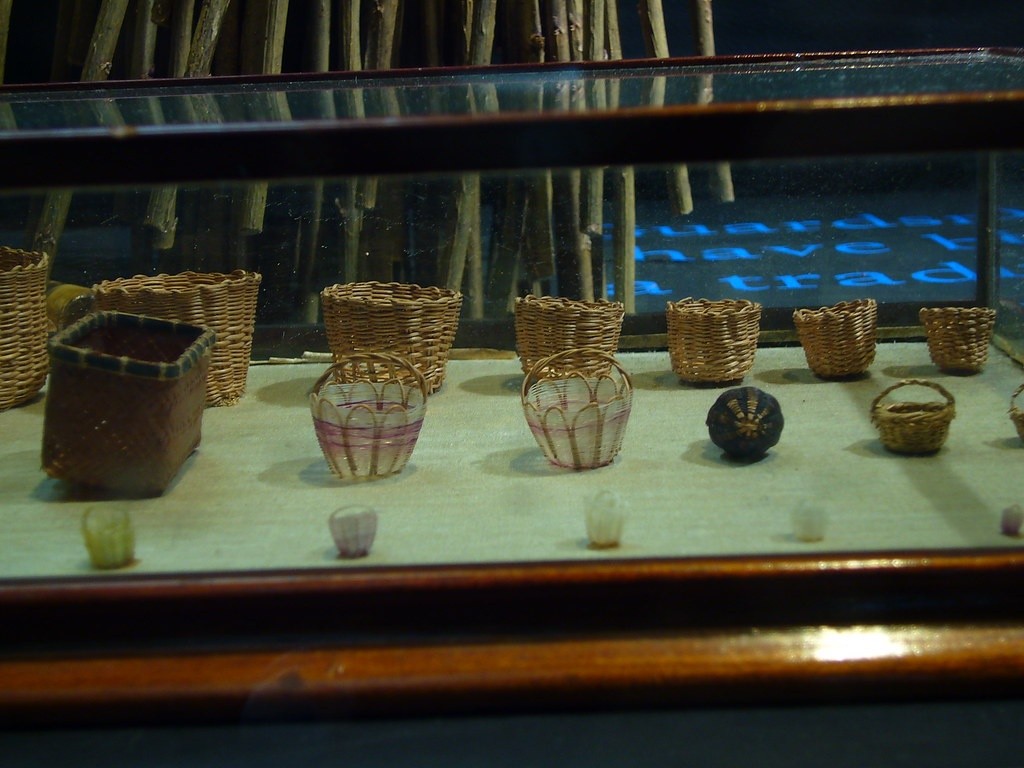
[0,246,48,412]
[308,353,427,480]
[41,310,216,498]
[514,294,625,381]
[92,271,262,407]
[870,379,956,454]
[919,307,997,373]
[1009,384,1024,442]
[792,299,877,378]
[523,348,634,469]
[665,297,762,383]
[321,281,464,395]
